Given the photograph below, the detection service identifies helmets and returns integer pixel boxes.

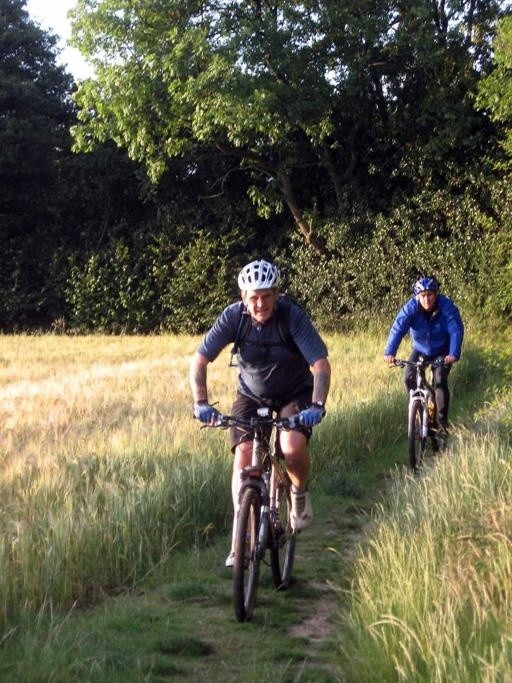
[237,259,280,291]
[413,277,437,295]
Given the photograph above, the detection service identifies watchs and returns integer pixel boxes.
[311,400,324,408]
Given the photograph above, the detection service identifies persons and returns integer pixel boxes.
[383,278,464,435]
[189,259,330,566]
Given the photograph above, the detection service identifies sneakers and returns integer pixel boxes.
[225,530,250,570]
[289,483,313,531]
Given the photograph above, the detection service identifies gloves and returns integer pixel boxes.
[300,405,326,427]
[195,400,220,423]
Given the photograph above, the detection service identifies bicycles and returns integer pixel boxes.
[194,402,327,622]
[387,354,452,477]
